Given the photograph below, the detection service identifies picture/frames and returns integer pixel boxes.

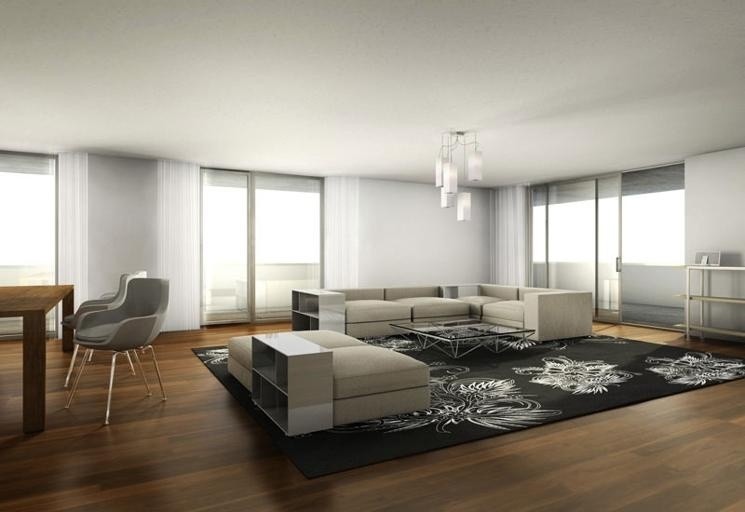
[692,250,722,267]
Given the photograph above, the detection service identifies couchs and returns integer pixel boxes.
[332,283,472,340]
[453,281,595,345]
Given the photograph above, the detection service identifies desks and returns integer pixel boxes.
[0,285,75,432]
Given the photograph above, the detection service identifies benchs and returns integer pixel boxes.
[229,328,430,424]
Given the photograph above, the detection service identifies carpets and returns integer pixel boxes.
[189,336,745,479]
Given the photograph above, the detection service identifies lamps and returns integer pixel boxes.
[434,129,484,225]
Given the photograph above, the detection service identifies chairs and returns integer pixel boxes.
[66,276,172,427]
[62,270,153,392]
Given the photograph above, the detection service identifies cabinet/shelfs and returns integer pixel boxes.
[252,335,333,439]
[673,264,744,350]
[291,288,346,333]
[438,283,482,300]
